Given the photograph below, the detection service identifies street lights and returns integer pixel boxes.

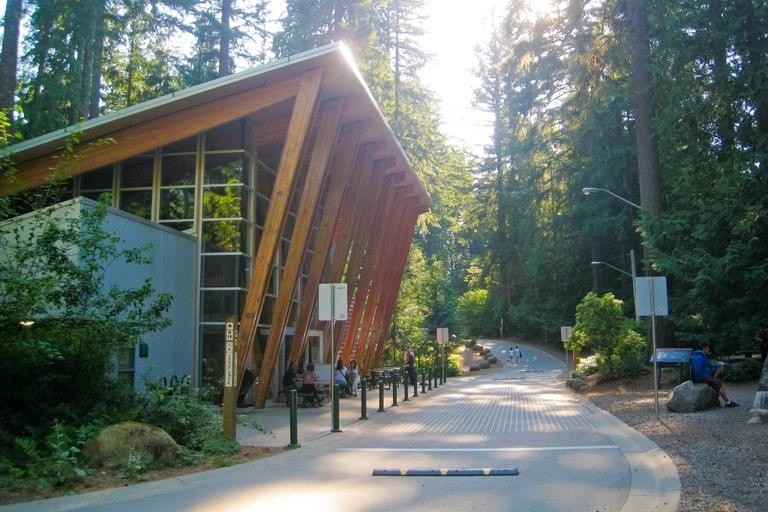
[590,250,640,324]
[583,188,643,211]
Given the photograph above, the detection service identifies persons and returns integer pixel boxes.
[514,345,521,365]
[691,341,741,408]
[302,363,317,392]
[404,346,415,368]
[348,359,360,396]
[284,361,300,392]
[335,358,347,386]
[508,348,513,363]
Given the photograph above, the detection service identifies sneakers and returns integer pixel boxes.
[725,401,740,407]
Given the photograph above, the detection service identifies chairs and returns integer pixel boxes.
[282,366,402,408]
